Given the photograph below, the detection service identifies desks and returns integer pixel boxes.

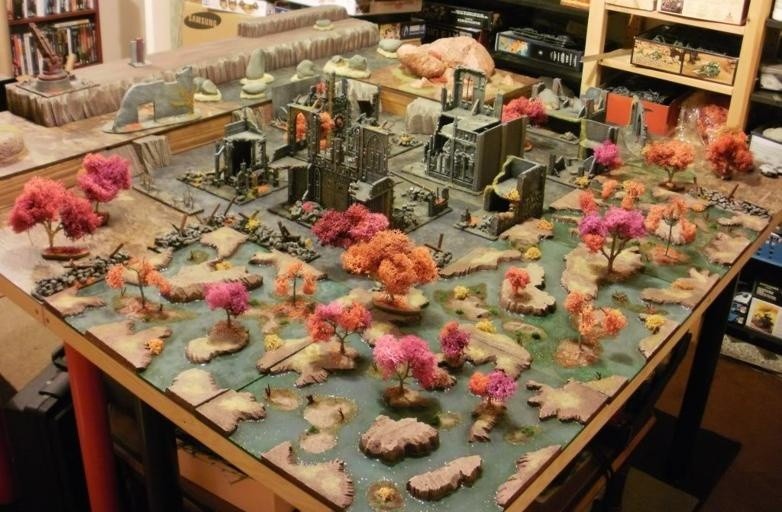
[1,6,780,510]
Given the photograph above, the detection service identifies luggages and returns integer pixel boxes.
[4,336,87,512]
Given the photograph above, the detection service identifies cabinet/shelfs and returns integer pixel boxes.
[420,0,782,374]
[5,2,104,78]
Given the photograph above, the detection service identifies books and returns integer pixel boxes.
[749,118,782,167]
[11,16,100,76]
[6,0,97,20]
